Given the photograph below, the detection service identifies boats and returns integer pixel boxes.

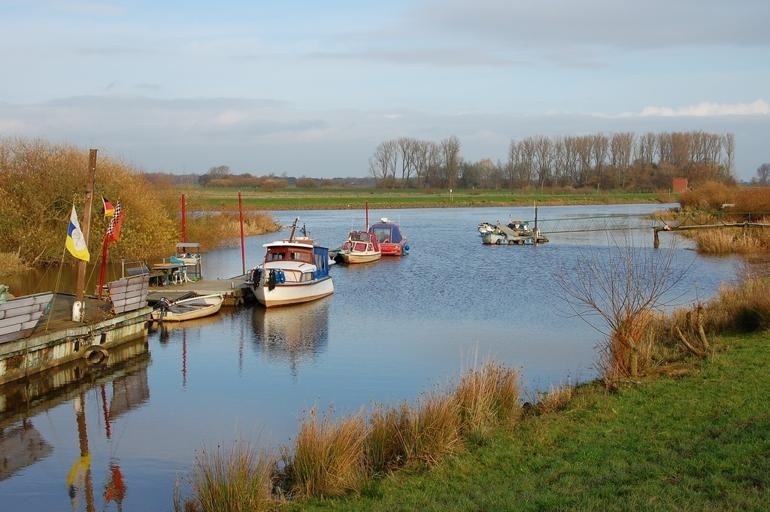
[244,218,409,307]
[478,221,549,245]
[150,294,224,322]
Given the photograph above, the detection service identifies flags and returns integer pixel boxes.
[102,462,129,510]
[109,202,124,240]
[67,450,91,510]
[101,197,115,217]
[66,205,91,262]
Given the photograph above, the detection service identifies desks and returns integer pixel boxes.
[152,266,182,285]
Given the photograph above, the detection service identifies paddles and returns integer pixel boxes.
[168,290,230,307]
